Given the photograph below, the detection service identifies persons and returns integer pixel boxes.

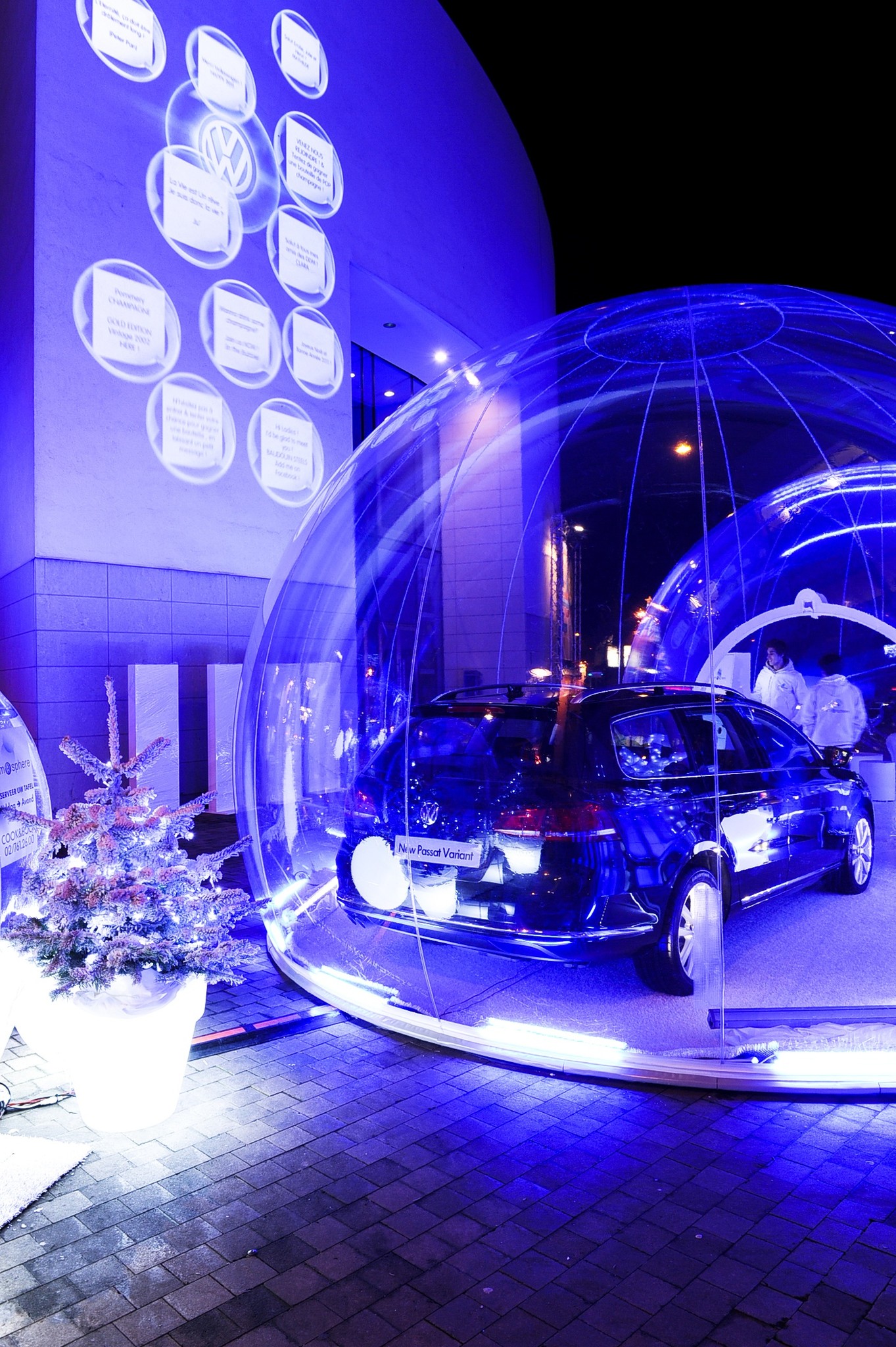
[801,653,867,770]
[752,639,808,728]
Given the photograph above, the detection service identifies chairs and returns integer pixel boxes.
[490,734,531,759]
[687,715,722,771]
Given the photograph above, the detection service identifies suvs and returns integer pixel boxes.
[335,684,876,998]
